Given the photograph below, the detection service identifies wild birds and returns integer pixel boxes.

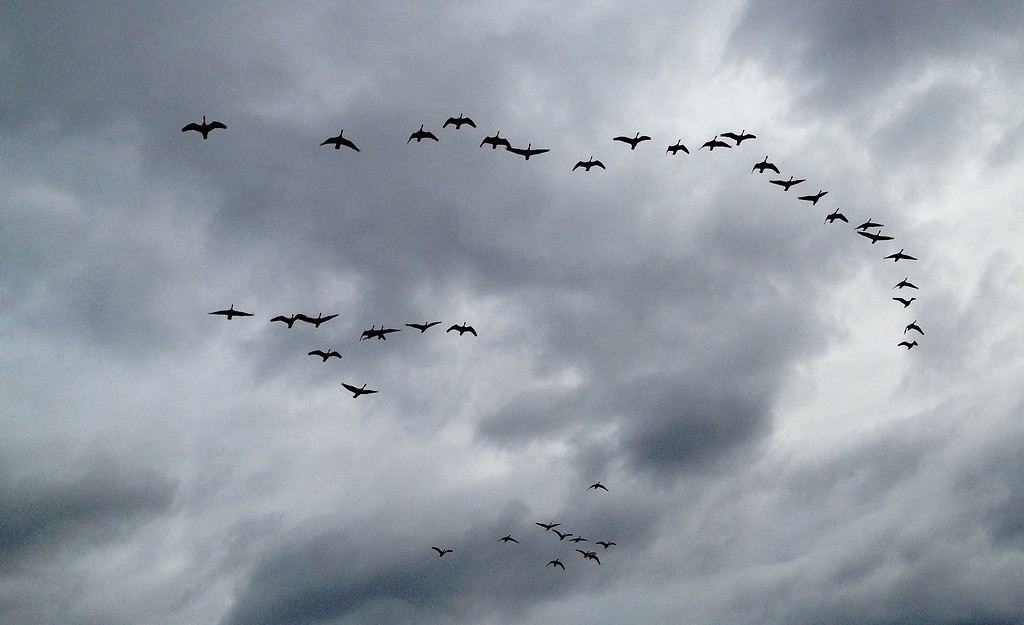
[535,522,616,572]
[443,113,476,131]
[699,129,756,151]
[407,124,439,143]
[497,535,519,544]
[432,547,453,557]
[665,139,689,156]
[206,304,478,400]
[319,129,360,152]
[572,155,606,171]
[613,132,651,150]
[750,156,918,262]
[480,131,550,160]
[181,115,227,139]
[586,482,609,492]
[891,278,924,350]
